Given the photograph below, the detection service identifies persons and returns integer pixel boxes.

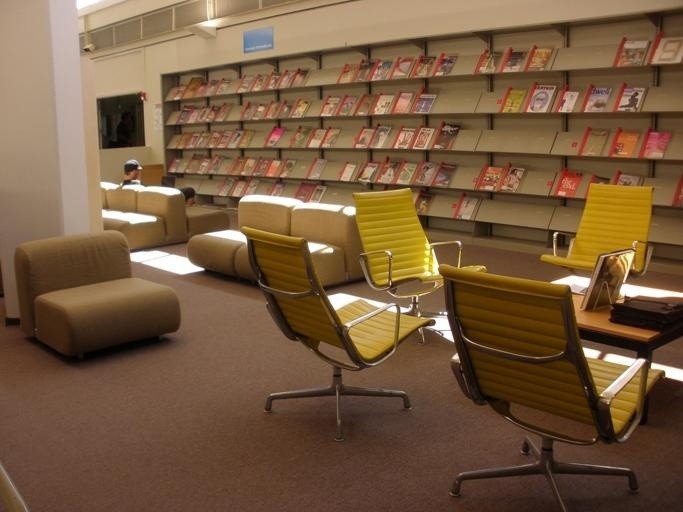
[122,159,144,185]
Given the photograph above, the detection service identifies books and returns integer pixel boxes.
[202,198,239,215]
[353,121,459,151]
[175,102,234,123]
[373,185,435,214]
[453,193,483,222]
[219,177,260,198]
[320,91,438,116]
[265,124,342,149]
[336,52,457,84]
[338,155,457,187]
[268,180,329,204]
[178,129,254,148]
[305,158,327,179]
[474,38,683,207]
[168,153,297,177]
[167,67,312,100]
[241,97,312,119]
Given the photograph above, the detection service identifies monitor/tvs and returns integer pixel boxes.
[96,94,144,149]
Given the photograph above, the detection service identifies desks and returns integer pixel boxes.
[544,271,682,376]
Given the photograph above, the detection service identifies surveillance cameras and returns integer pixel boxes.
[83,44,96,52]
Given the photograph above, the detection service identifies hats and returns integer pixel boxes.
[123,159,143,172]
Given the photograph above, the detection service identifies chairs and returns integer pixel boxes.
[434,260,666,503]
[12,228,182,360]
[348,186,488,344]
[537,182,654,282]
[240,222,436,441]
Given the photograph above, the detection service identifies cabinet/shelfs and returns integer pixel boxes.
[155,7,681,272]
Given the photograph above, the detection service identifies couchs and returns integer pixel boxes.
[99,180,232,249]
[185,192,366,292]
